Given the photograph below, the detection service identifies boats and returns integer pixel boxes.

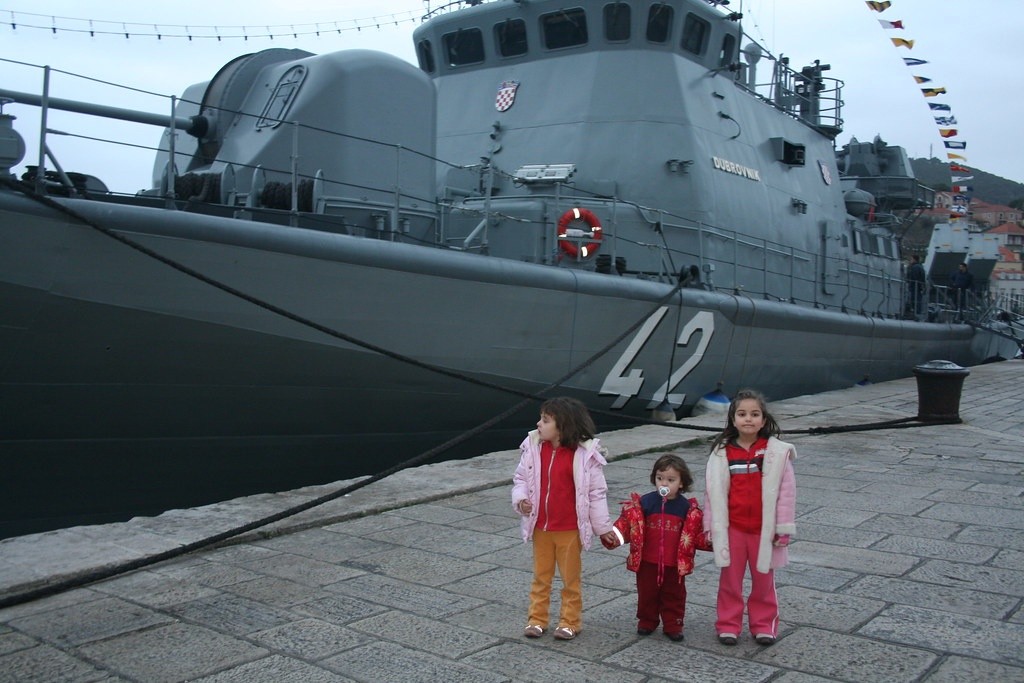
[0,1,1024,539]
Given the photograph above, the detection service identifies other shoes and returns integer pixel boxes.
[756,634,773,644]
[554,627,577,639]
[667,632,683,641]
[719,632,737,644]
[638,628,651,635]
[524,624,542,637]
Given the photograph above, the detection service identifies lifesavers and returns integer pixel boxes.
[556,207,602,259]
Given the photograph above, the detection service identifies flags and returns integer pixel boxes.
[866,0,892,13]
[890,38,914,49]
[939,129,957,138]
[950,165,970,175]
[921,87,946,97]
[878,19,904,29]
[953,186,974,192]
[949,194,974,220]
[928,102,951,111]
[934,115,957,126]
[944,141,966,149]
[903,58,927,66]
[914,76,931,83]
[947,153,966,161]
[951,176,974,183]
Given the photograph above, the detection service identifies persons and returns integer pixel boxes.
[601,453,713,641]
[509,395,614,639]
[950,262,975,308]
[907,254,926,313]
[706,389,796,644]
